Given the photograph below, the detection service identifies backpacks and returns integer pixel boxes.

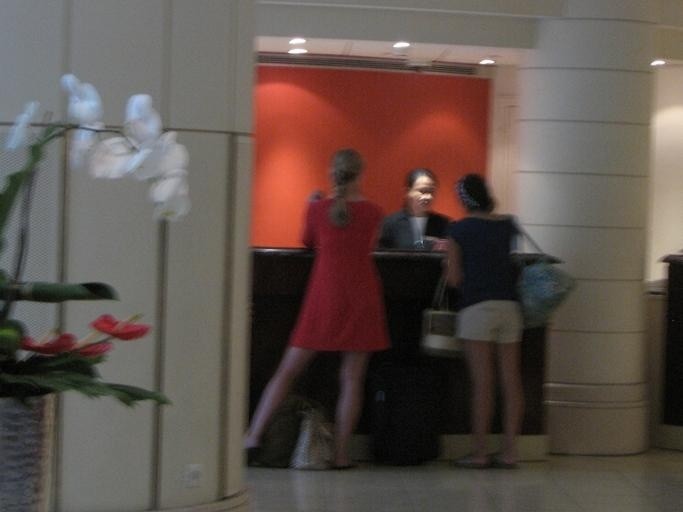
[365,387,446,470]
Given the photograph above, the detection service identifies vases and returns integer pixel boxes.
[0,393,57,512]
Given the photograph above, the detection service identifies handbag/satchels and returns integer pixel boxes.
[287,409,338,471]
[420,307,465,359]
[256,393,306,468]
[503,253,580,330]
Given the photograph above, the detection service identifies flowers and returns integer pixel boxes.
[0,73,190,409]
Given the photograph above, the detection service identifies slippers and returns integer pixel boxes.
[245,424,266,466]
[453,453,523,469]
[333,454,358,470]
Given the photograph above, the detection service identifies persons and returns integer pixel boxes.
[375,168,456,251]
[439,171,527,469]
[239,147,391,470]
